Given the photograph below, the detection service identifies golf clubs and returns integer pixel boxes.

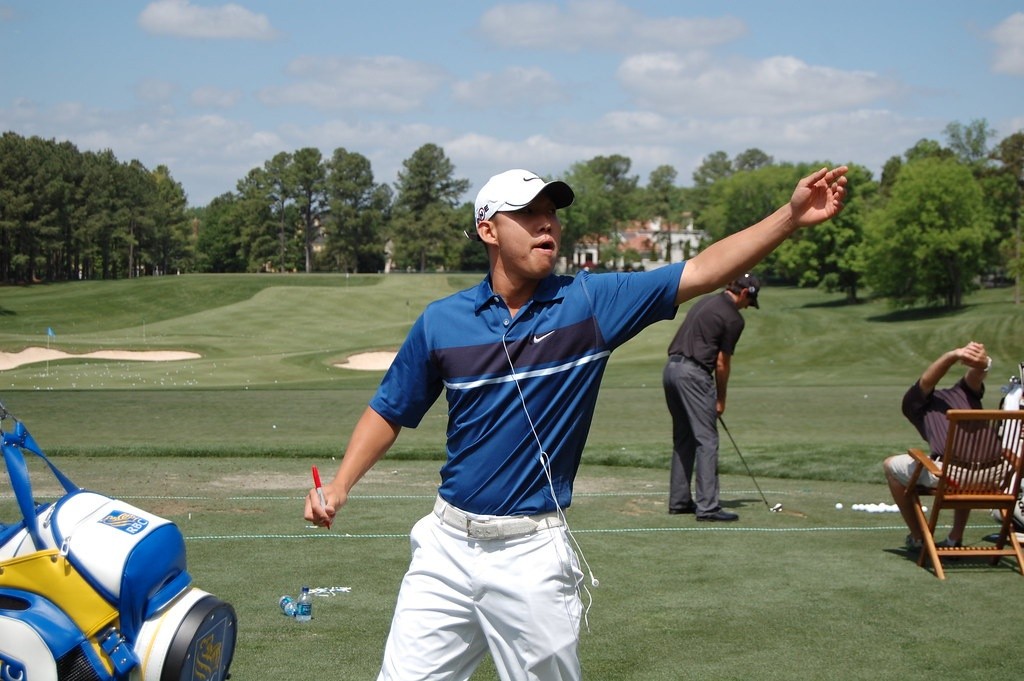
[718,416,782,512]
[272,425,277,428]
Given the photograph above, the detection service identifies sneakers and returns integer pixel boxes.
[935,540,961,547]
[905,533,924,551]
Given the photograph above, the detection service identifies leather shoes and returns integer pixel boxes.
[668,501,697,514]
[695,508,739,522]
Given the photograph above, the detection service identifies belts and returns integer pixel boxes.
[432,494,563,539]
[930,455,1003,470]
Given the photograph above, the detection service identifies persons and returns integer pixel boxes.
[302,166,849,681]
[662,272,761,522]
[883,341,992,550]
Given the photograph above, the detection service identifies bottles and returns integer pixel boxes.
[296,587,312,622]
[279,595,298,617]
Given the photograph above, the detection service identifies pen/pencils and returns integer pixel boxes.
[312,465,331,531]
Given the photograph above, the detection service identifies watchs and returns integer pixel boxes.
[977,356,992,373]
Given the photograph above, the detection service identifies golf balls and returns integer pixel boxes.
[1,363,250,390]
[922,506,928,512]
[851,502,901,513]
[835,503,843,510]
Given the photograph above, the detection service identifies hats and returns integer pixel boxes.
[464,168,576,241]
[736,272,761,309]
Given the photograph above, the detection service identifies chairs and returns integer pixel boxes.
[903,409,1024,581]
